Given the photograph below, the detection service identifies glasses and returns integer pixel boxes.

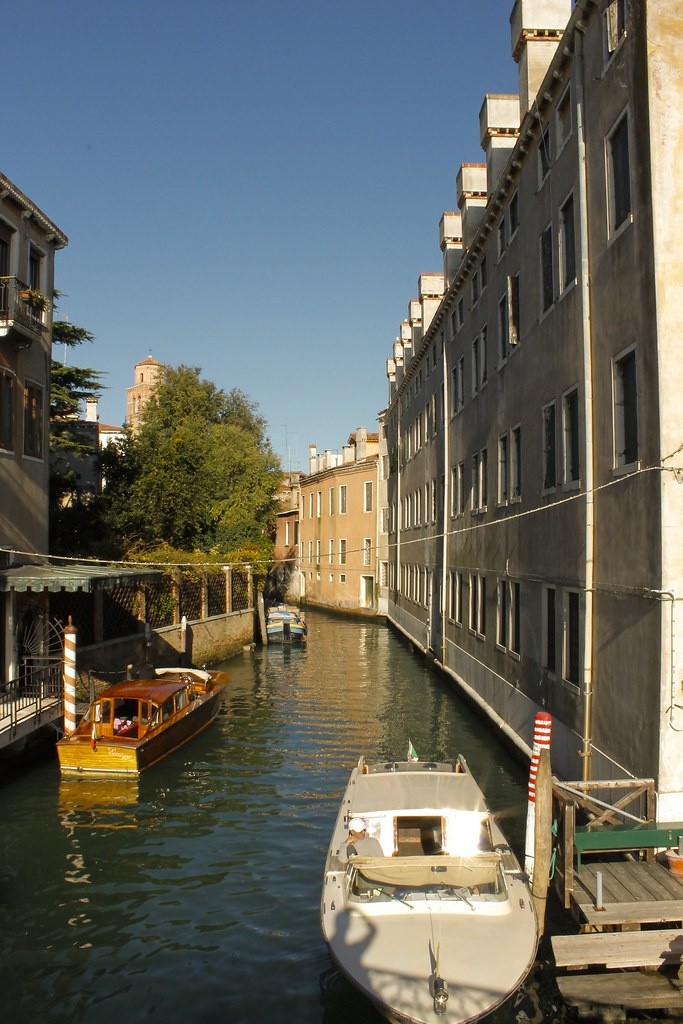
[359,829,367,833]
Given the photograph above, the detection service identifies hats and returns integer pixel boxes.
[349,818,369,833]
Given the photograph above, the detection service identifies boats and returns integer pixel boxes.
[321,754,539,1024]
[56,666,230,775]
[266,604,309,644]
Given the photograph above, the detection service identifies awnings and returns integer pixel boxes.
[0,563,165,593]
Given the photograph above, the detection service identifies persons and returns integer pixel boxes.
[113,709,127,731]
[338,818,385,863]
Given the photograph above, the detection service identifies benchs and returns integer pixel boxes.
[574,821,683,875]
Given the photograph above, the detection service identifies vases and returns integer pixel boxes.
[664,848,683,875]
